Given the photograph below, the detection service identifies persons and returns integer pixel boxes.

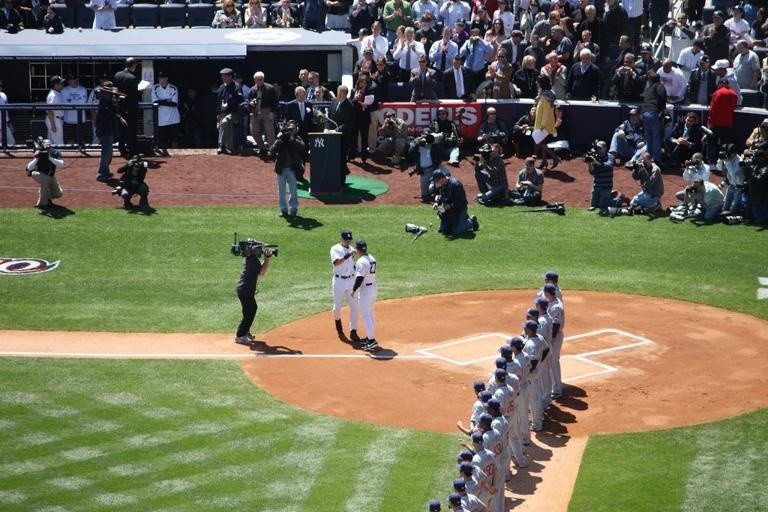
[1,0,65,34]
[91,0,118,29]
[210,0,767,236]
[350,240,378,350]
[330,230,361,342]
[235,242,273,346]
[0,92,16,149]
[24,57,204,211]
[421,272,565,512]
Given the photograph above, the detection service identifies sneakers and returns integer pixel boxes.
[335,319,378,351]
[246,331,255,339]
[469,215,478,231]
[234,337,251,344]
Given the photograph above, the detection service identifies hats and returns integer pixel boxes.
[710,58,729,69]
[219,68,233,74]
[41,139,51,148]
[596,141,606,148]
[510,30,525,38]
[428,169,443,182]
[429,272,562,511]
[50,75,66,84]
[340,230,352,240]
[711,10,722,16]
[639,46,651,53]
[355,240,367,250]
[364,47,372,53]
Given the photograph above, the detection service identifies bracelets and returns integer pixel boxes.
[344,253,350,260]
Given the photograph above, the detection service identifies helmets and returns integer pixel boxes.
[437,107,448,117]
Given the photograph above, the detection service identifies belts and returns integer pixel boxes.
[335,274,354,279]
[56,116,65,120]
[366,283,372,286]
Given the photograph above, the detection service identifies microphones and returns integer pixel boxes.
[321,115,339,131]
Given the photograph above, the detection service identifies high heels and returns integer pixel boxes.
[550,157,561,169]
[536,161,547,169]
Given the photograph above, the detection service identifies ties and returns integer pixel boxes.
[300,102,304,121]
[440,43,445,72]
[456,69,461,98]
[406,44,410,72]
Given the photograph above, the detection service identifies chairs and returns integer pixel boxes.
[48,0,304,27]
[735,84,763,108]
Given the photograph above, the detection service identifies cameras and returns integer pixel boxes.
[631,159,644,169]
[620,68,627,72]
[686,184,698,194]
[132,153,144,168]
[386,113,393,129]
[685,159,698,169]
